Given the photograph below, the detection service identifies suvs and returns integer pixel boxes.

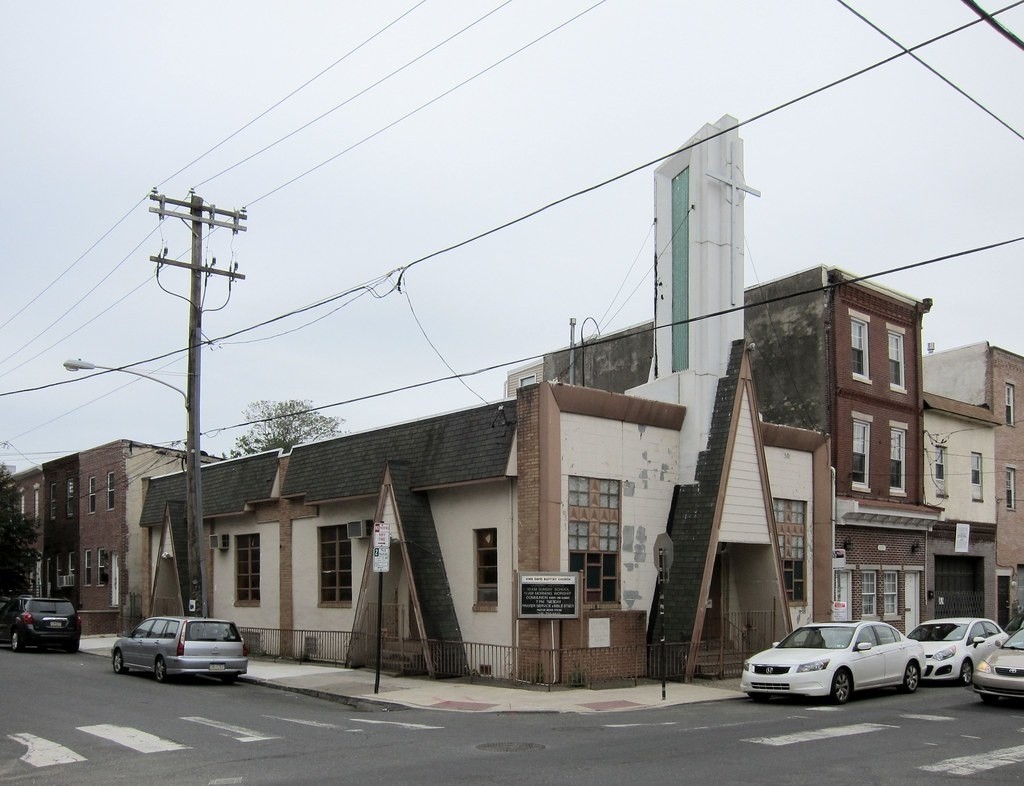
[0,594,82,653]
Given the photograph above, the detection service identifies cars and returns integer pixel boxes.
[111,614,248,684]
[906,618,1009,685]
[971,627,1024,702]
[739,620,927,705]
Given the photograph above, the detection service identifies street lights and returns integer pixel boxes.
[64,358,210,613]
